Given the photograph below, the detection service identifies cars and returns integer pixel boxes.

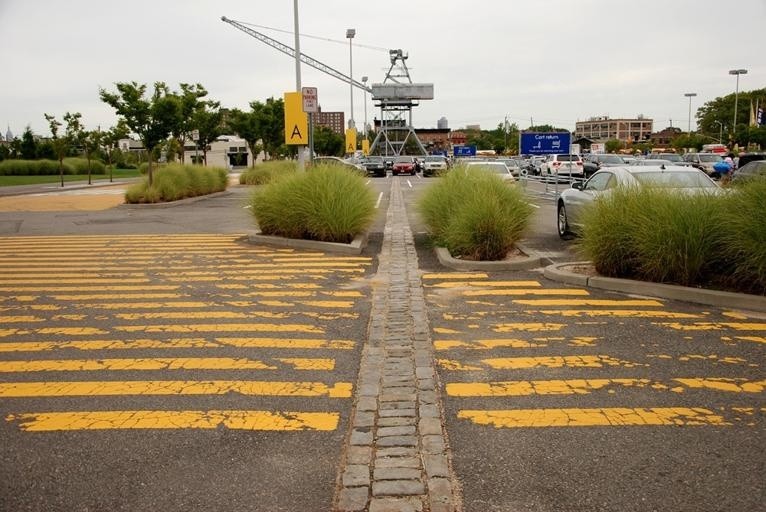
[539,154,584,180]
[555,161,733,243]
[584,151,732,176]
[313,155,362,174]
[458,154,549,182]
[422,155,448,177]
[363,156,389,177]
[392,155,417,176]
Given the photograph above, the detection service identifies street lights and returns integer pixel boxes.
[361,76,369,140]
[345,28,356,158]
[684,93,697,139]
[728,68,747,134]
